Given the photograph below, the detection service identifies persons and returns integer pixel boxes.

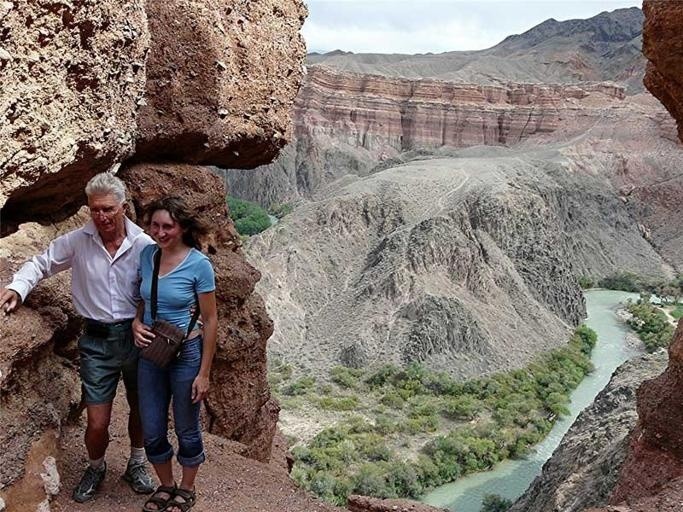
[1,174,202,504]
[133,196,219,512]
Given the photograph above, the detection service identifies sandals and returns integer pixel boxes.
[166,484,196,512]
[143,480,177,512]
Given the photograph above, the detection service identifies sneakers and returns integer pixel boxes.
[73,461,107,502]
[124,458,155,494]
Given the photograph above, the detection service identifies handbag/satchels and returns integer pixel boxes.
[138,320,186,369]
